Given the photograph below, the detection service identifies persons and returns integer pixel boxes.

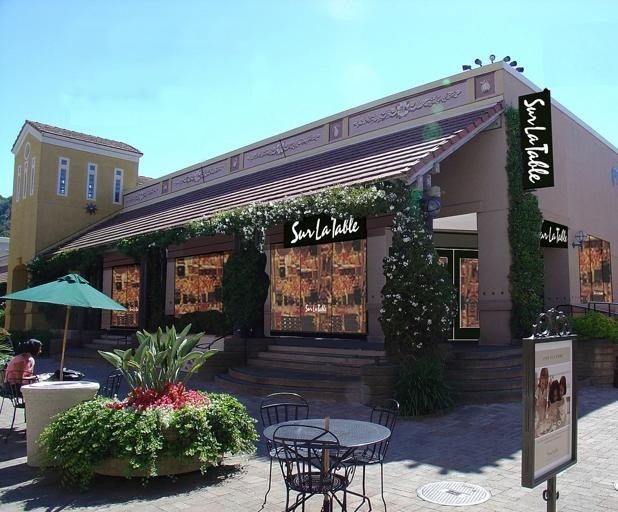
[0,337,43,398]
[537,366,570,436]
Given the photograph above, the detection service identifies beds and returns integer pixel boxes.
[0,273,130,380]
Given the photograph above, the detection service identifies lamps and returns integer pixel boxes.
[97,370,123,399]
[1,367,40,445]
[257,392,399,512]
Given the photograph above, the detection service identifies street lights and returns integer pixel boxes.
[54,367,85,380]
